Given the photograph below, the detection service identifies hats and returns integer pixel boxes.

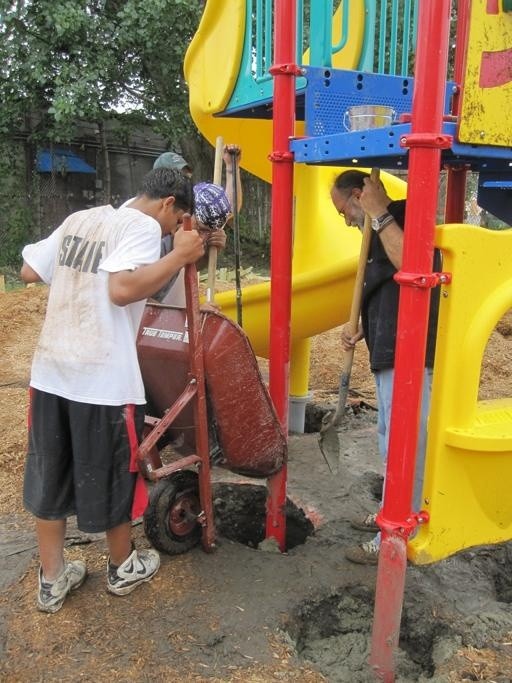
[153,152,192,170]
[192,179,233,231]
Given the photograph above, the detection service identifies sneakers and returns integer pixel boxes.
[35,557,87,613]
[104,541,160,597]
[346,541,379,567]
[350,512,382,534]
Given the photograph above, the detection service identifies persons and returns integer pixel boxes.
[331,169,445,568]
[20,162,198,612]
[140,180,231,306]
[112,142,244,220]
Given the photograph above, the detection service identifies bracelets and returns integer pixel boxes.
[375,219,396,235]
[225,167,240,173]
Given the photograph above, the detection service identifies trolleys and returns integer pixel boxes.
[136,213,288,556]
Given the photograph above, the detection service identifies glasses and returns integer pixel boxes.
[194,213,213,236]
[339,190,354,217]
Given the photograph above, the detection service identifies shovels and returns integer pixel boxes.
[316,167,380,475]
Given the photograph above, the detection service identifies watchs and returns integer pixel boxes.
[369,212,393,231]
